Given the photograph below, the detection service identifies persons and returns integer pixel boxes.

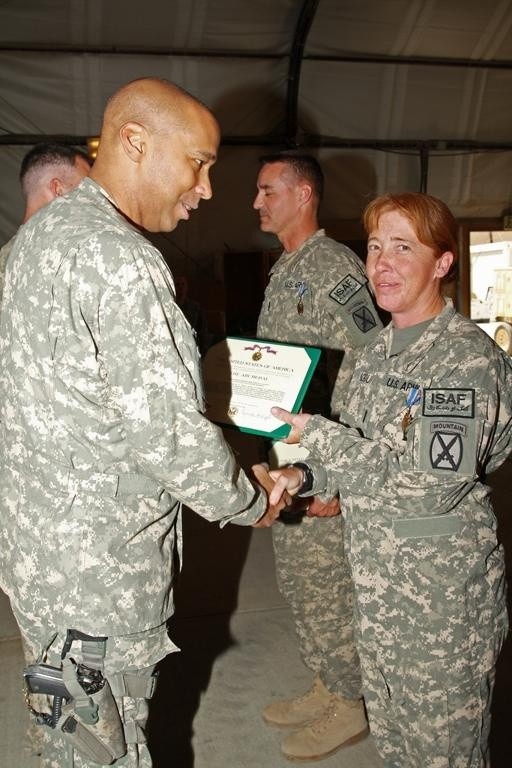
[2,77,291,767]
[244,153,385,762]
[2,146,106,287]
[268,187,511,768]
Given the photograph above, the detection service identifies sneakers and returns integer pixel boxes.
[260,675,371,762]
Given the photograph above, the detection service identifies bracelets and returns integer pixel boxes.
[284,462,313,497]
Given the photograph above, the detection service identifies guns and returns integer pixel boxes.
[24,664,105,703]
